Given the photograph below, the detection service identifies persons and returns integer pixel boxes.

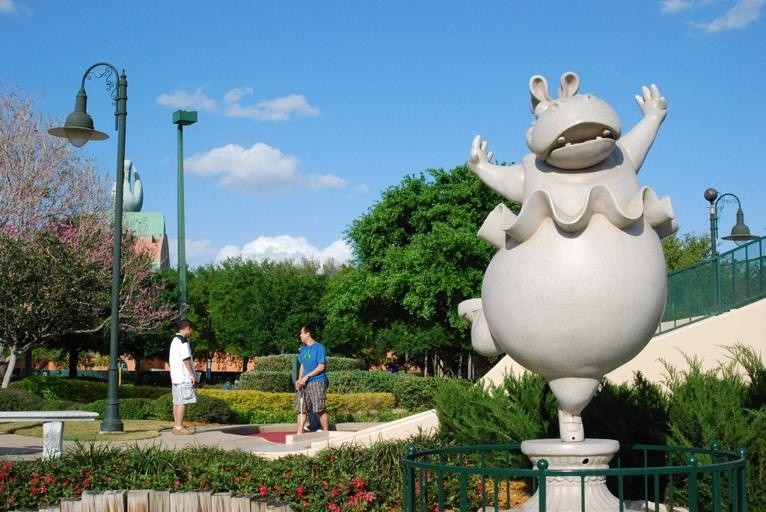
[169,319,197,435]
[234,375,240,389]
[293,323,329,435]
[223,378,232,390]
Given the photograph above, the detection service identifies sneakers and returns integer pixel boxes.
[171,426,197,435]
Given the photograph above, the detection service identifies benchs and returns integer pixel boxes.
[0,410,100,462]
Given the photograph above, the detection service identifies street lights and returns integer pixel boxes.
[49,64,126,430]
[704,189,759,257]
[172,110,197,318]
